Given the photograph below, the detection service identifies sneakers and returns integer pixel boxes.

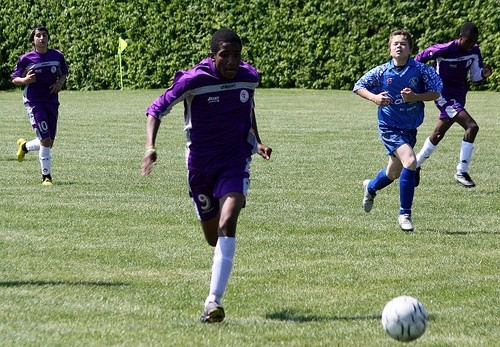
[454,171,475,187]
[41,177,53,187]
[414,166,421,187]
[397,213,413,231]
[362,179,376,213]
[16,138,28,162]
[200,301,226,323]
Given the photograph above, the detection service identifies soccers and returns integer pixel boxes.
[381,295,427,342]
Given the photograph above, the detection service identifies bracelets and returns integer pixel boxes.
[144,146,157,155]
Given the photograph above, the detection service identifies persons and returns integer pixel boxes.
[141,29,273,324]
[11,27,68,185]
[414,21,493,188]
[353,30,443,231]
